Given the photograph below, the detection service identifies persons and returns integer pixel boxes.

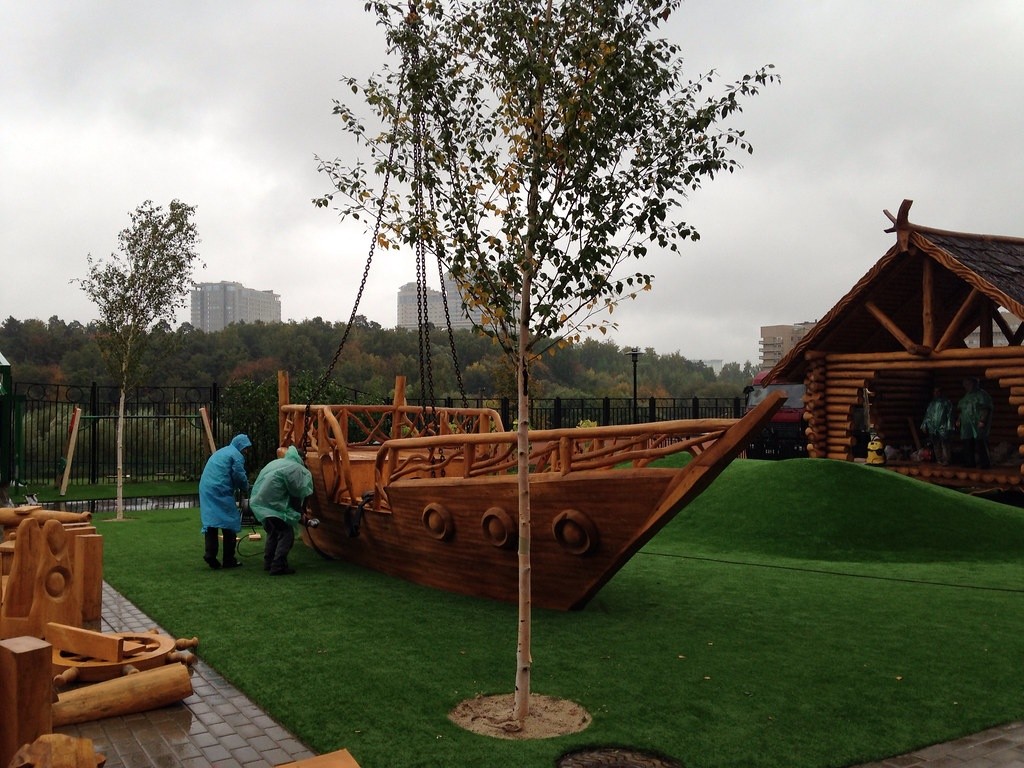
[957,375,994,468]
[198,434,251,569]
[248,446,312,576]
[920,386,957,465]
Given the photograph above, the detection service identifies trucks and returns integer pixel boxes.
[740,369,808,460]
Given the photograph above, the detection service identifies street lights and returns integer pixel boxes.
[624,347,644,425]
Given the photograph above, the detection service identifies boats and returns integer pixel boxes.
[273,366,792,615]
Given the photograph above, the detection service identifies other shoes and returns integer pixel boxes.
[265,570,296,576]
[204,556,222,570]
[223,562,242,568]
